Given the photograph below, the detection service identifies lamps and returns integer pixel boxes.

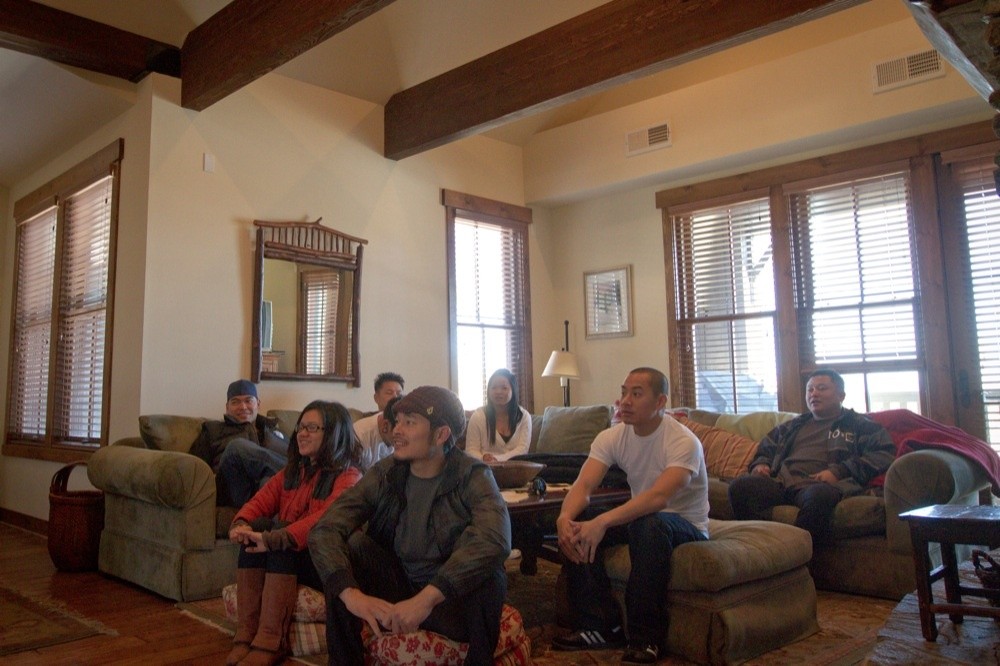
[541,351,582,407]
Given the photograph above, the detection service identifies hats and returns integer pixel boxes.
[390,387,468,442]
[226,379,258,401]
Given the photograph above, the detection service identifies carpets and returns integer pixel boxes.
[181,584,894,664]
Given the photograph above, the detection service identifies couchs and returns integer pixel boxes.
[86,435,241,603]
[519,407,995,602]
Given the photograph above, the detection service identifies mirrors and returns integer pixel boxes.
[249,215,370,387]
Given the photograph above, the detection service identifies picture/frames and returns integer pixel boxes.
[583,263,633,340]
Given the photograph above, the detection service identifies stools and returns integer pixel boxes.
[356,603,532,666]
[563,515,823,665]
[222,579,329,656]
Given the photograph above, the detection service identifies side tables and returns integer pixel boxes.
[896,501,1000,641]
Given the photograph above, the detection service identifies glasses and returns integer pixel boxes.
[295,422,325,433]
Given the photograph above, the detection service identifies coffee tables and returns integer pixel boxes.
[492,476,633,583]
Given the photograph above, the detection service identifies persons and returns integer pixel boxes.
[727,368,897,581]
[222,401,367,666]
[356,373,406,420]
[188,379,292,511]
[351,395,406,470]
[550,367,711,666]
[307,386,513,666]
[465,369,533,464]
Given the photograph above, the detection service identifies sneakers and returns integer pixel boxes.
[620,640,668,666]
[551,628,624,646]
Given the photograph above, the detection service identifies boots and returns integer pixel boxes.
[226,568,270,665]
[237,572,299,666]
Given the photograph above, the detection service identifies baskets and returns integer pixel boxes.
[973,549,999,624]
[48,459,104,570]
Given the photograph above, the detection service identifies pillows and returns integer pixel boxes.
[532,404,610,457]
[137,413,209,453]
